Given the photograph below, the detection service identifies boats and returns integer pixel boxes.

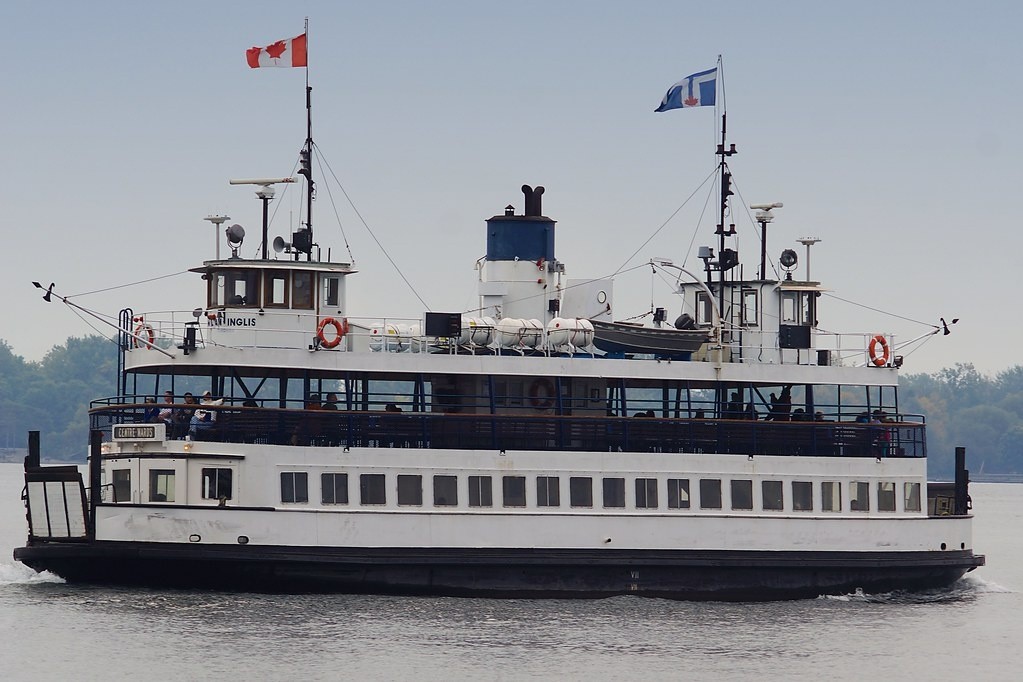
[574,315,712,356]
[11,86,986,607]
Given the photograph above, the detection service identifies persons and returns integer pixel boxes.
[158,391,176,426]
[188,391,229,440]
[694,388,824,454]
[171,392,197,440]
[291,394,345,446]
[143,397,160,423]
[856,410,894,457]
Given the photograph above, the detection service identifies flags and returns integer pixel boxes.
[654,67,718,112]
[246,32,306,68]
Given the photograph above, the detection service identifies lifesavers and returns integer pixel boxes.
[317,318,343,350]
[134,325,154,350]
[869,336,890,365]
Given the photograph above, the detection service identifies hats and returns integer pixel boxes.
[203,391,211,396]
